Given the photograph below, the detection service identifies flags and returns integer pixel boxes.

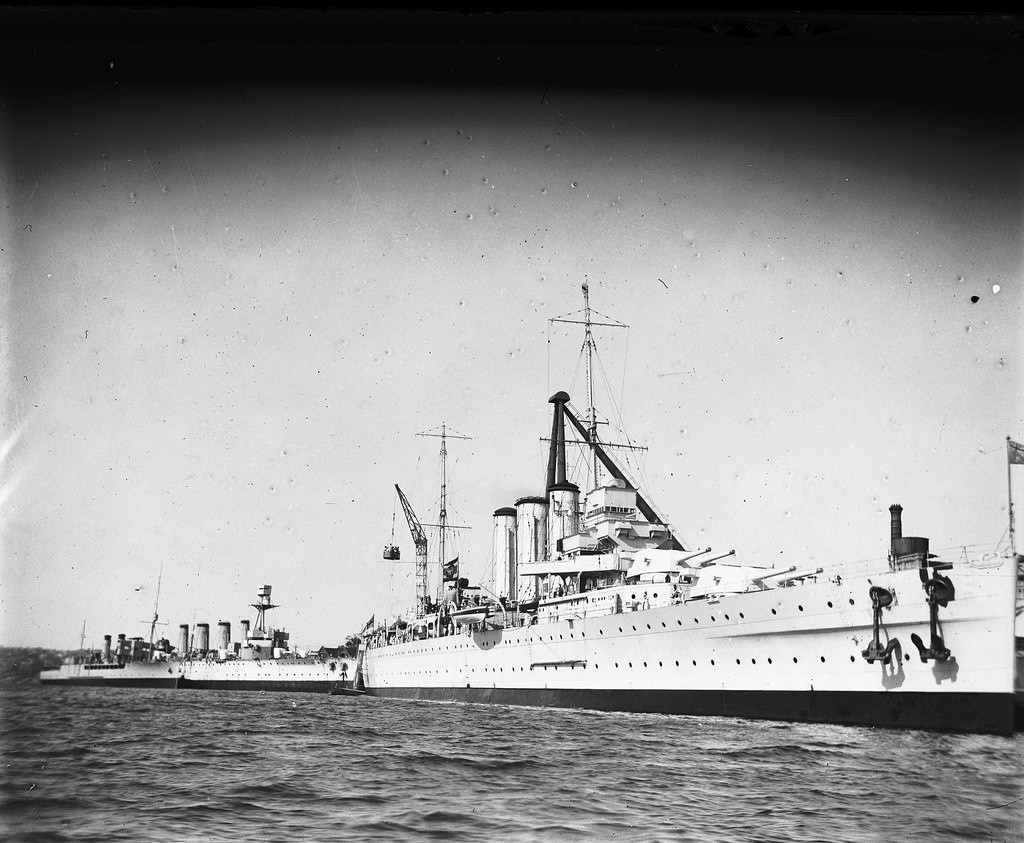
[366,616,374,630]
[1008,440,1024,465]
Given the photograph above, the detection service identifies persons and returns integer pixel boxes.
[610,595,615,614]
[643,591,650,610]
[553,584,564,598]
[671,583,684,605]
[631,594,642,612]
[557,555,562,561]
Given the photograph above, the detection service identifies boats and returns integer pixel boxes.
[35,558,365,693]
[360,272,1024,738]
